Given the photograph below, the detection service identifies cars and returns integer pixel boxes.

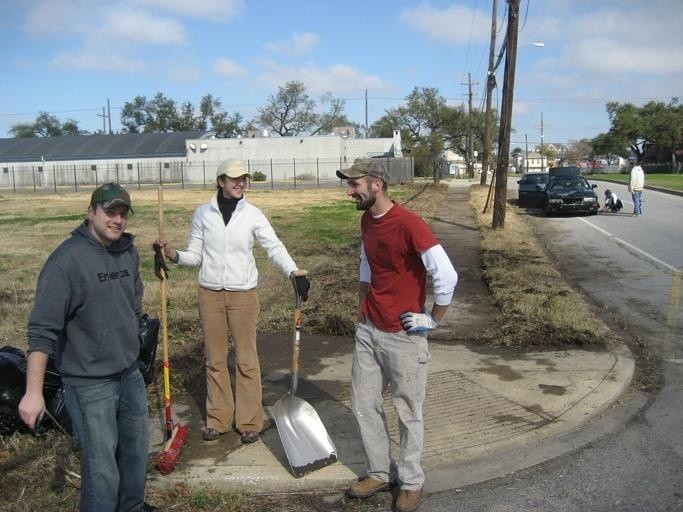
[535,166,600,217]
[477,169,481,174]
[516,172,550,204]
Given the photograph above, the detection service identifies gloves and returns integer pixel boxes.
[152,243,169,280]
[291,271,311,302]
[400,306,439,335]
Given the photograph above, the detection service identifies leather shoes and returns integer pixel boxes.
[395,484,423,512]
[348,474,396,498]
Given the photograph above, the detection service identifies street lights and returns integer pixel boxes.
[479,42,544,186]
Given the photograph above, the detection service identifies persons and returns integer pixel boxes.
[601,189,623,213]
[628,159,645,215]
[336,160,458,512]
[18,182,159,511]
[154,159,310,443]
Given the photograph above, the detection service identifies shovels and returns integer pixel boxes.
[273,271,337,479]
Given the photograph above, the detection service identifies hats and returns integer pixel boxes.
[90,182,134,215]
[215,158,253,181]
[337,159,391,185]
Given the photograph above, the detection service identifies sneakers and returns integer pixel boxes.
[241,430,259,443]
[203,426,219,441]
